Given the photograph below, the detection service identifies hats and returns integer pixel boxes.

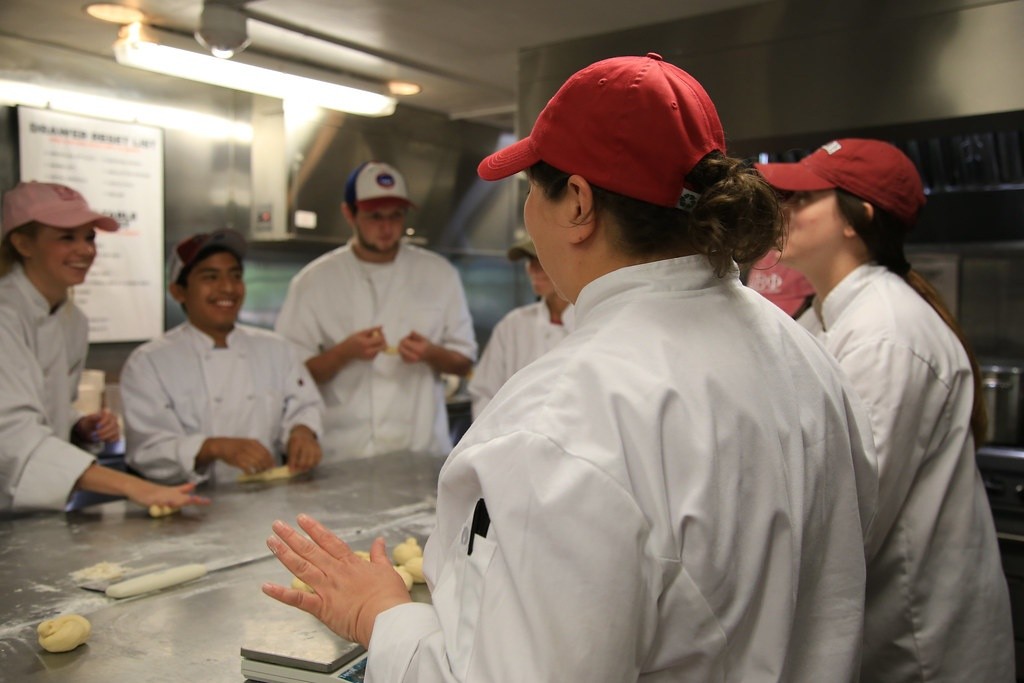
[746,247,817,318]
[477,52,726,213]
[346,161,419,211]
[754,139,926,232]
[168,229,247,284]
[510,239,538,261]
[2,181,118,239]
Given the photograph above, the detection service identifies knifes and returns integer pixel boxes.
[107,551,273,597]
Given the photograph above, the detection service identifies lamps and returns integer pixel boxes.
[112,24,398,117]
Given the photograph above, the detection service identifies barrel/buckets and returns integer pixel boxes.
[978,366,1022,445]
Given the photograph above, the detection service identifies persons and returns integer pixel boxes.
[0,163,813,519]
[263,54,880,683]
[754,139,1017,683]
